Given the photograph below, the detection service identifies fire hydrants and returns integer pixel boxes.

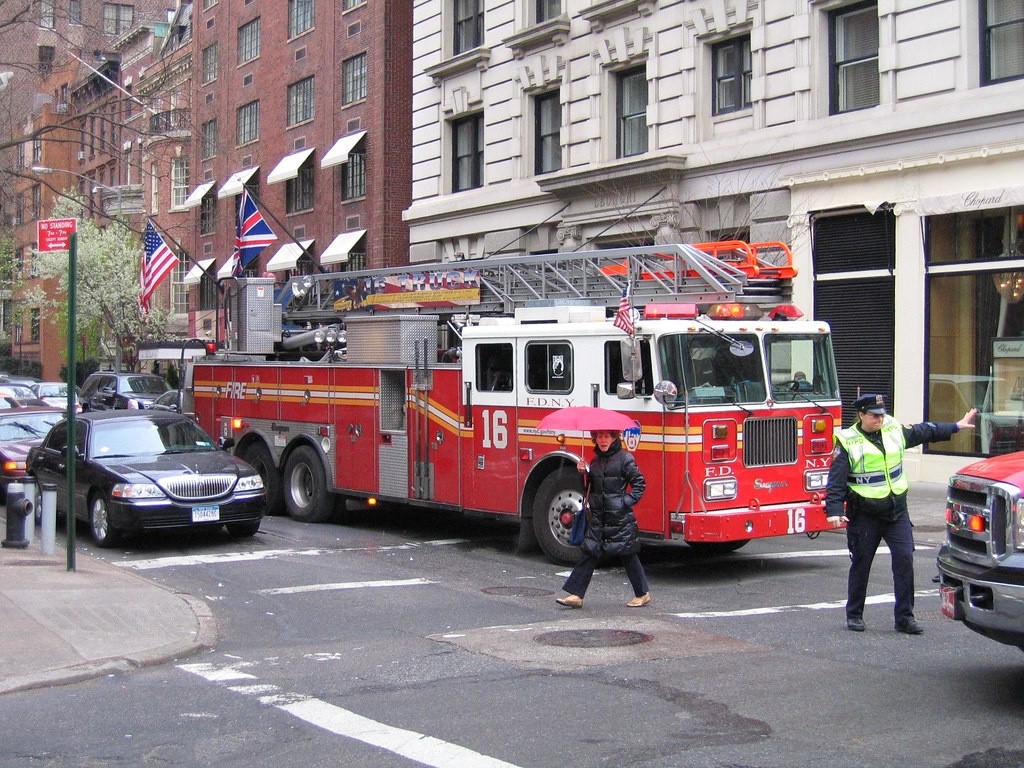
[0,480,33,548]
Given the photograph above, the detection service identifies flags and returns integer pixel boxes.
[139,220,180,310]
[613,286,633,334]
[232,188,279,275]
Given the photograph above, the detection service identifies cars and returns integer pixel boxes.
[25,409,267,548]
[0,372,50,410]
[0,405,68,506]
[144,389,185,412]
[29,382,85,415]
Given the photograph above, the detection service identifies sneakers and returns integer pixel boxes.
[847,617,865,631]
[893,619,923,632]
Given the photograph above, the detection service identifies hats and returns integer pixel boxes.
[852,393,887,414]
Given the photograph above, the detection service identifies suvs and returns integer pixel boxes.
[930,449,1024,652]
[76,371,172,413]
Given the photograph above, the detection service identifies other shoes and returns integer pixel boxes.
[555,597,583,609]
[627,592,652,608]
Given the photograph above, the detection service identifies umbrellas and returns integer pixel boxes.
[537,405,639,460]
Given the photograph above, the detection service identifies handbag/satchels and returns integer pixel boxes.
[568,480,590,547]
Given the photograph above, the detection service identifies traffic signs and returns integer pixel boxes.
[37,218,78,251]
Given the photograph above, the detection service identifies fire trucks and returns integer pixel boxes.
[179,241,850,567]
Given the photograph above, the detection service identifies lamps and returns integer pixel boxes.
[987,241,1024,302]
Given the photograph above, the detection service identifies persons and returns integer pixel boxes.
[791,371,812,389]
[556,430,651,607]
[826,394,977,631]
[167,365,178,387]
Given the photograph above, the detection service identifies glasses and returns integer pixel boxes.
[866,413,885,419]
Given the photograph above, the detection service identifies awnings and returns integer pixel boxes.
[267,240,316,272]
[320,229,367,266]
[218,166,260,199]
[185,258,216,285]
[186,180,216,208]
[321,131,366,169]
[268,146,316,185]
[218,253,240,278]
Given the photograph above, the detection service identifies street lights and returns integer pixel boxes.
[31,167,123,249]
